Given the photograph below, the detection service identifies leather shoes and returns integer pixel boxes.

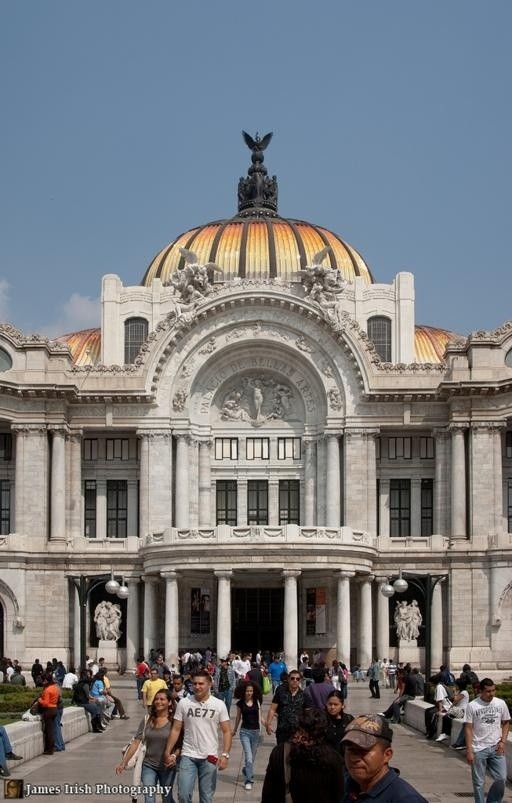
[5,752,23,760]
[0,768,10,776]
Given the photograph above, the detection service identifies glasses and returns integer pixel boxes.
[291,677,301,682]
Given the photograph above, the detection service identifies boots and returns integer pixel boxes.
[91,714,105,733]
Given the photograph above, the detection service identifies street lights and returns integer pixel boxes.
[75,569,131,674]
[381,573,448,682]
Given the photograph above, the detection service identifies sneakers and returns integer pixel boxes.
[450,744,467,750]
[435,734,449,741]
[245,784,251,790]
[377,713,385,717]
[120,716,130,720]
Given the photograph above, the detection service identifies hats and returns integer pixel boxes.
[339,714,393,749]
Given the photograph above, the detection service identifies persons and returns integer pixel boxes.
[2,645,511,803]
[161,262,213,322]
[95,600,108,638]
[223,377,292,418]
[409,599,422,639]
[201,594,210,611]
[394,600,412,639]
[242,131,272,173]
[298,262,347,316]
[105,601,122,640]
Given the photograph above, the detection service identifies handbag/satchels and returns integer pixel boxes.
[30,702,42,715]
[121,736,143,770]
[263,676,270,694]
[106,695,114,703]
[258,733,265,743]
